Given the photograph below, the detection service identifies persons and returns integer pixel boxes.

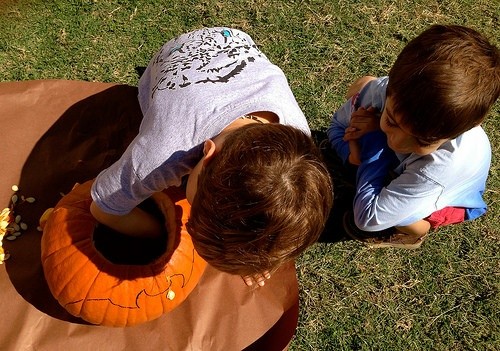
[325,24,500,250]
[90,26,334,288]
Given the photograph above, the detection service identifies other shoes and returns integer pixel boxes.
[343,209,429,250]
[320,138,355,176]
